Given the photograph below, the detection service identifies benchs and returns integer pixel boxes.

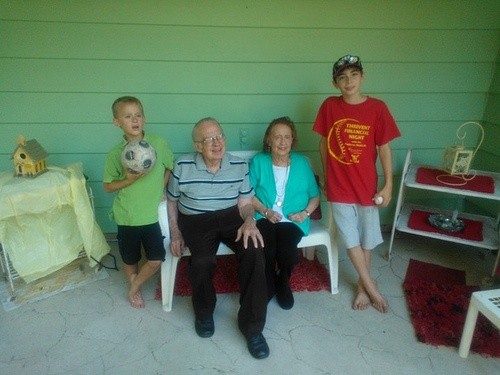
[157,151,340,312]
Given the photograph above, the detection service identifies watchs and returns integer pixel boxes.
[249,216,257,222]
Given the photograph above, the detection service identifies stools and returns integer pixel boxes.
[458,289,500,359]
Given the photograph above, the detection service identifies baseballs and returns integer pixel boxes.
[374,196,383,205]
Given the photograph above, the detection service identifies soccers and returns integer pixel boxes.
[121,139,157,174]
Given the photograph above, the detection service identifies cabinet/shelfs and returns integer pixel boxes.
[0,165,103,293]
[389,148,500,277]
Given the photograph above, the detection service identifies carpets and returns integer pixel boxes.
[154,255,330,300]
[402,257,500,358]
[0,255,109,311]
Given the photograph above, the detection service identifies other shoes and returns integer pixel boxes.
[275,290,294,310]
[246,332,272,359]
[195,314,217,337]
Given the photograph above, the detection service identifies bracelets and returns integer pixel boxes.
[305,210,310,216]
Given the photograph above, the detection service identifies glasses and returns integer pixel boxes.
[335,56,359,67]
[196,135,224,145]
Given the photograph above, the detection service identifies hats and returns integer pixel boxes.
[332,55,364,79]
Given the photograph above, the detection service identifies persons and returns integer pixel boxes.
[249,117,321,310]
[102,96,175,308]
[165,118,270,359]
[313,55,401,314]
